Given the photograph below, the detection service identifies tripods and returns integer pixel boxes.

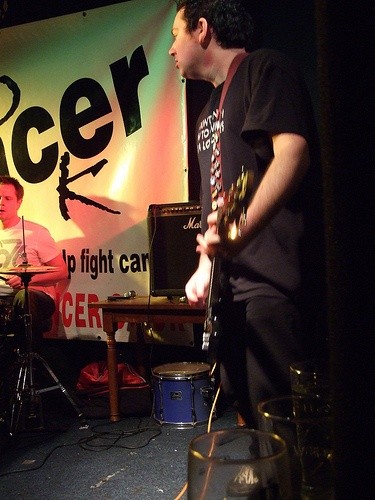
[6,274,90,449]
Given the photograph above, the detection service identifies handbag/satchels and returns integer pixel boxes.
[75,362,153,416]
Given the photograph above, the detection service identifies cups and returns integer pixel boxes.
[187,428,293,500]
[257,395,339,500]
[290,359,340,487]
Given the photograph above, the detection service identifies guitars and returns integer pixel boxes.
[202,165,254,353]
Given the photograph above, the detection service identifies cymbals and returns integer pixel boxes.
[0,266,59,274]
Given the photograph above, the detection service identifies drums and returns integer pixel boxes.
[151,361,217,427]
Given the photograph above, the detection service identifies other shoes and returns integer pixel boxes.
[26,407,40,430]
[224,465,261,500]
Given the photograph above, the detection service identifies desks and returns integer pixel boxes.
[88,296,210,424]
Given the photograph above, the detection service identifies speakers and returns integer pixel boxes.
[147,201,202,298]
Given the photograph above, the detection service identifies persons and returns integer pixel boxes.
[168,0,328,500]
[0,176,69,362]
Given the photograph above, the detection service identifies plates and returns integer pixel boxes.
[109,296,130,299]
